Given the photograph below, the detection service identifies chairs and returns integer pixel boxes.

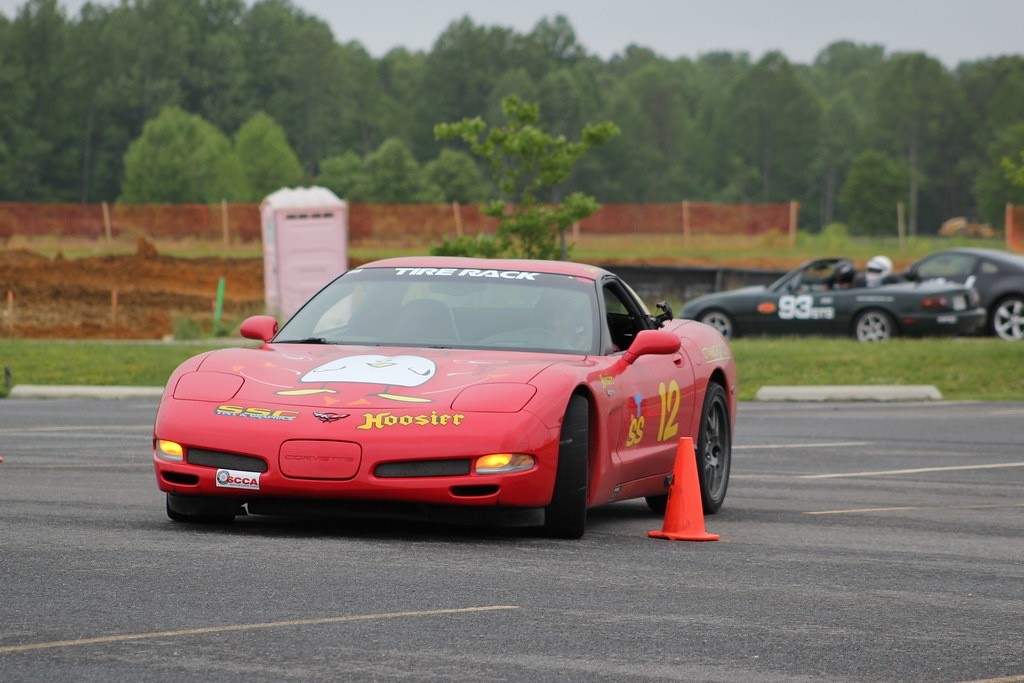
[850,272,865,289]
[387,297,460,344]
[537,288,593,348]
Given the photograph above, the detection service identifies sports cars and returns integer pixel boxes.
[677,255,987,344]
[150,248,740,538]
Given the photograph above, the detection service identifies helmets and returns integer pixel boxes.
[833,263,857,284]
[864,254,893,288]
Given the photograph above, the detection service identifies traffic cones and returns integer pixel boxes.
[645,437,721,542]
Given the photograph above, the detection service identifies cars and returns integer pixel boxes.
[897,244,1024,341]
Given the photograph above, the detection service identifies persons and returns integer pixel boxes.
[865,255,893,288]
[821,261,856,290]
